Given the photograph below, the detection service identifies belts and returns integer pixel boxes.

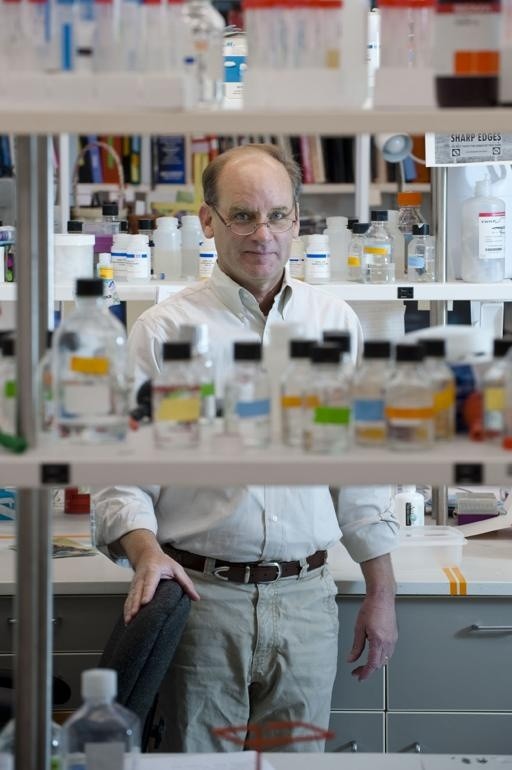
[159,542,327,585]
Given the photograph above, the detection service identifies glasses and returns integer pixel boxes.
[212,202,298,236]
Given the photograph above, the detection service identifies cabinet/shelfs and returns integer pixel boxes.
[0,107,512,769]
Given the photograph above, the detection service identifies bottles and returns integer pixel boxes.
[393,482,426,529]
[57,667,144,770]
[1,0,439,110]
[1,279,510,449]
[1,178,438,286]
[1,712,66,770]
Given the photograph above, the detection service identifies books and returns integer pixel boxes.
[84,134,351,188]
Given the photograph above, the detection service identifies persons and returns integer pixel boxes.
[91,144,399,755]
